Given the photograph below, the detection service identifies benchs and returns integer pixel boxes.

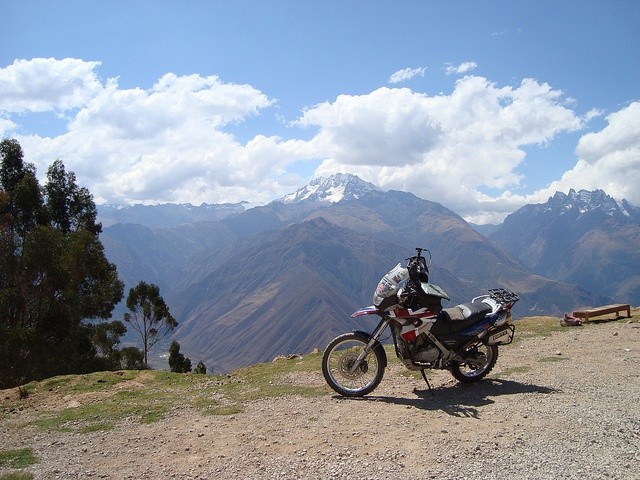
[572,303,631,325]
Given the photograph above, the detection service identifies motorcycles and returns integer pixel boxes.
[322,247,519,397]
